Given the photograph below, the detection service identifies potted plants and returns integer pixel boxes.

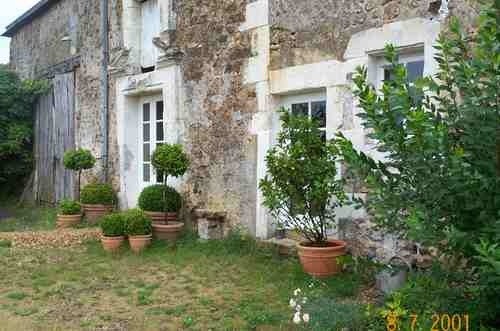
[99,213,125,251]
[55,199,83,229]
[148,143,190,242]
[257,105,348,277]
[80,181,114,222]
[125,215,153,252]
[138,182,182,221]
